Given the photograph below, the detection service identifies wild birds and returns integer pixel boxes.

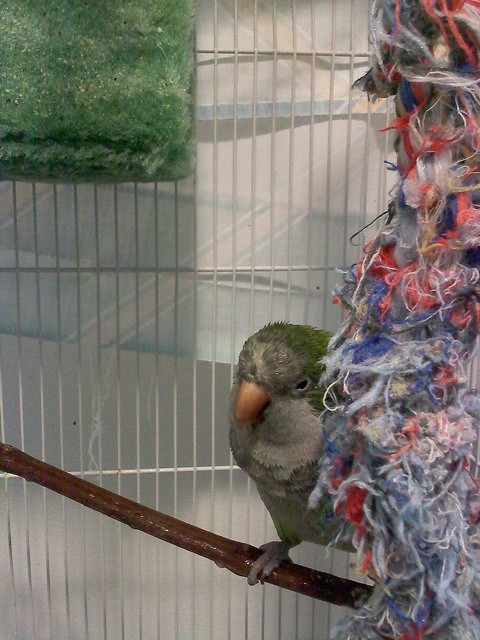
[227,320,376,589]
